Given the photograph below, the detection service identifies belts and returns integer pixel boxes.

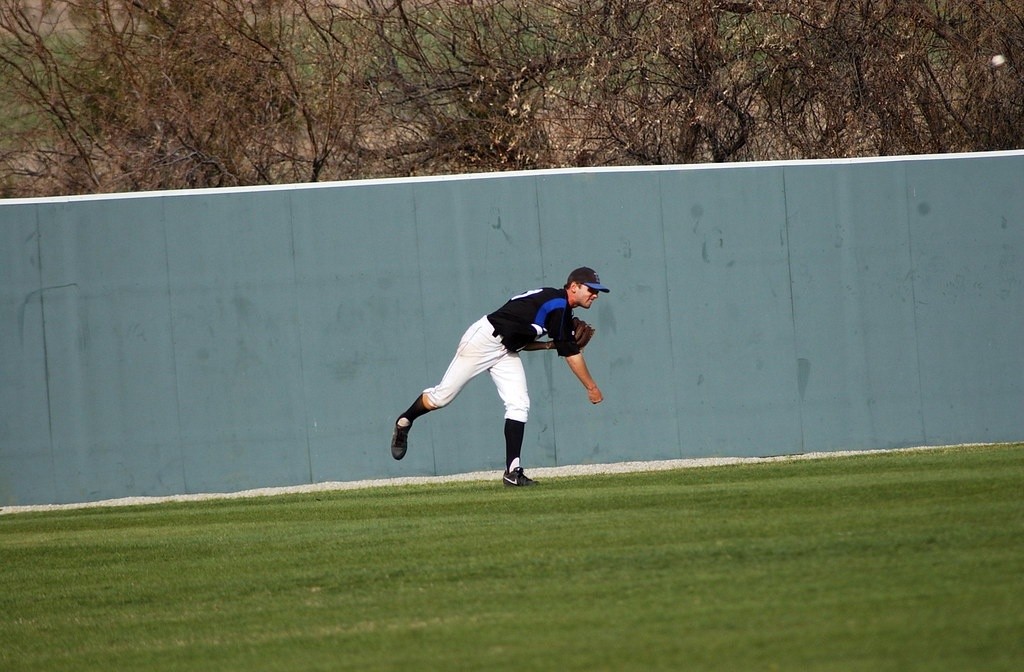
[493,331,509,350]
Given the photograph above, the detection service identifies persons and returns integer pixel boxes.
[392,267,610,486]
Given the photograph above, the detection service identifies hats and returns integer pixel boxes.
[567,267,610,293]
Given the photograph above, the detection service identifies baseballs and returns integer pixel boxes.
[993,55,1004,67]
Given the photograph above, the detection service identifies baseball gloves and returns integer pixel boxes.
[571,314,593,350]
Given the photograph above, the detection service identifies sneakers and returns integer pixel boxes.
[503,467,540,487]
[391,414,412,460]
